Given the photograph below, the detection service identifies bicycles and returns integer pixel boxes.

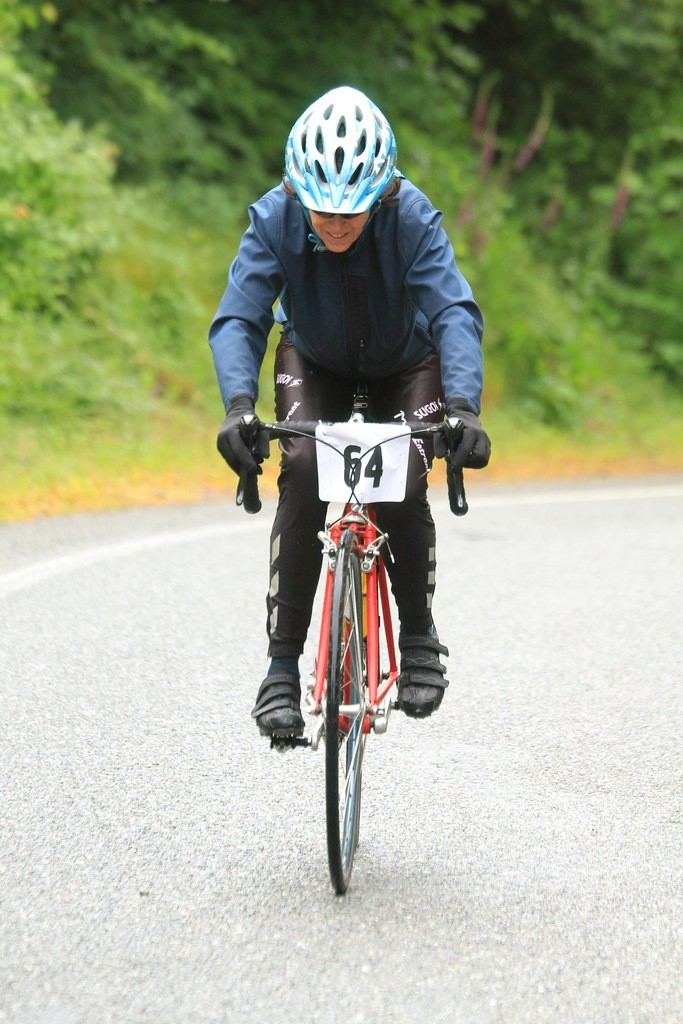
[233,411,470,896]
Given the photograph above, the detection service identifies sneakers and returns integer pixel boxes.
[250,672,304,737]
[398,623,449,719]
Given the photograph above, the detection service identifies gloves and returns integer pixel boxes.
[216,398,269,478]
[432,397,491,474]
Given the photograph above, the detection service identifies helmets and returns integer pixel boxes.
[284,86,396,214]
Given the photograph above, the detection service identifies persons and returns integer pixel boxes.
[207,85,492,741]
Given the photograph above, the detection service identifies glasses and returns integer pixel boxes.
[312,210,361,219]
